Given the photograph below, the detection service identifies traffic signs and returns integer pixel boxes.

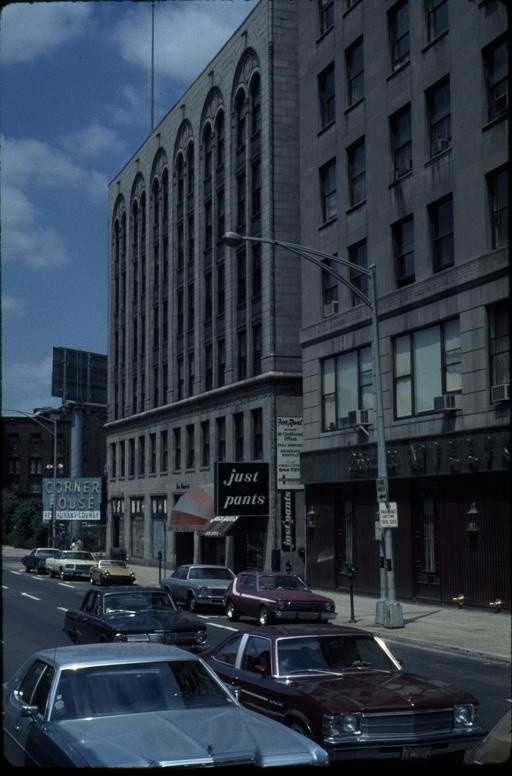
[375,476,387,503]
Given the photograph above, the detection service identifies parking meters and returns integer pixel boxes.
[346,563,359,623]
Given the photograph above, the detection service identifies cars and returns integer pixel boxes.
[19,547,137,588]
[222,569,338,627]
[59,585,210,657]
[158,562,238,617]
[2,639,331,769]
[199,618,492,771]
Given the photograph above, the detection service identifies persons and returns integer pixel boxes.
[287,646,327,671]
[258,649,274,678]
[71,538,82,551]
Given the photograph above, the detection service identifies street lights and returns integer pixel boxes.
[219,226,406,633]
[43,463,66,548]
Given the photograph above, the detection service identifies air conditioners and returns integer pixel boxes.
[324,301,338,317]
[491,383,510,403]
[434,394,463,412]
[431,139,449,154]
[348,410,373,426]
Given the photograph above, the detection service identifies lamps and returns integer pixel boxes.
[465,503,480,533]
[306,504,317,528]
